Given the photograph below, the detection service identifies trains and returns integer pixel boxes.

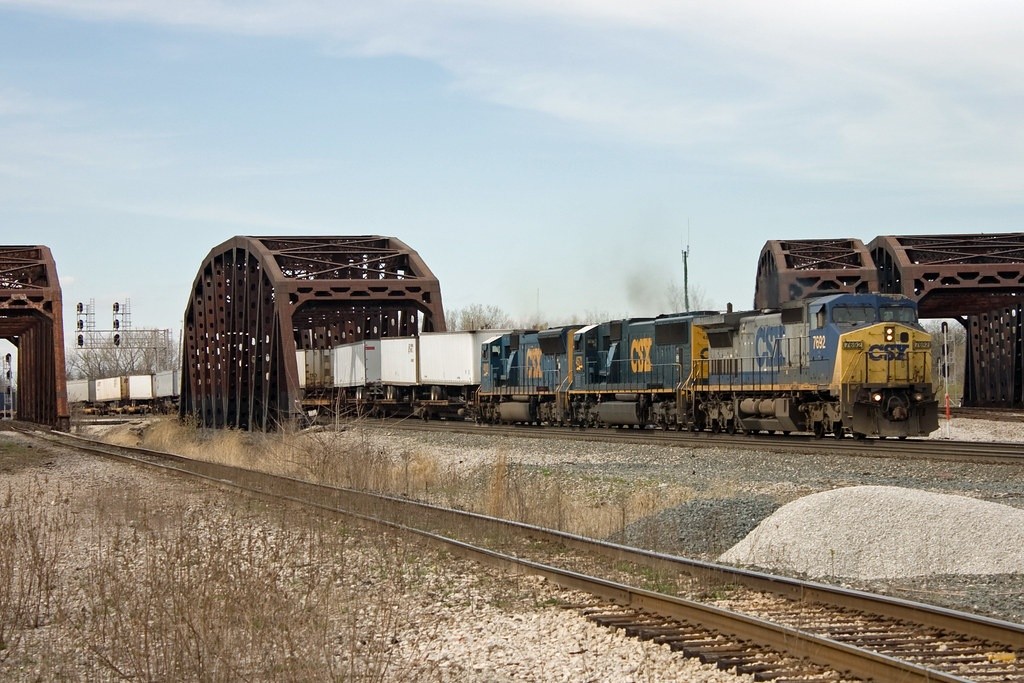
[66,292,942,441]
